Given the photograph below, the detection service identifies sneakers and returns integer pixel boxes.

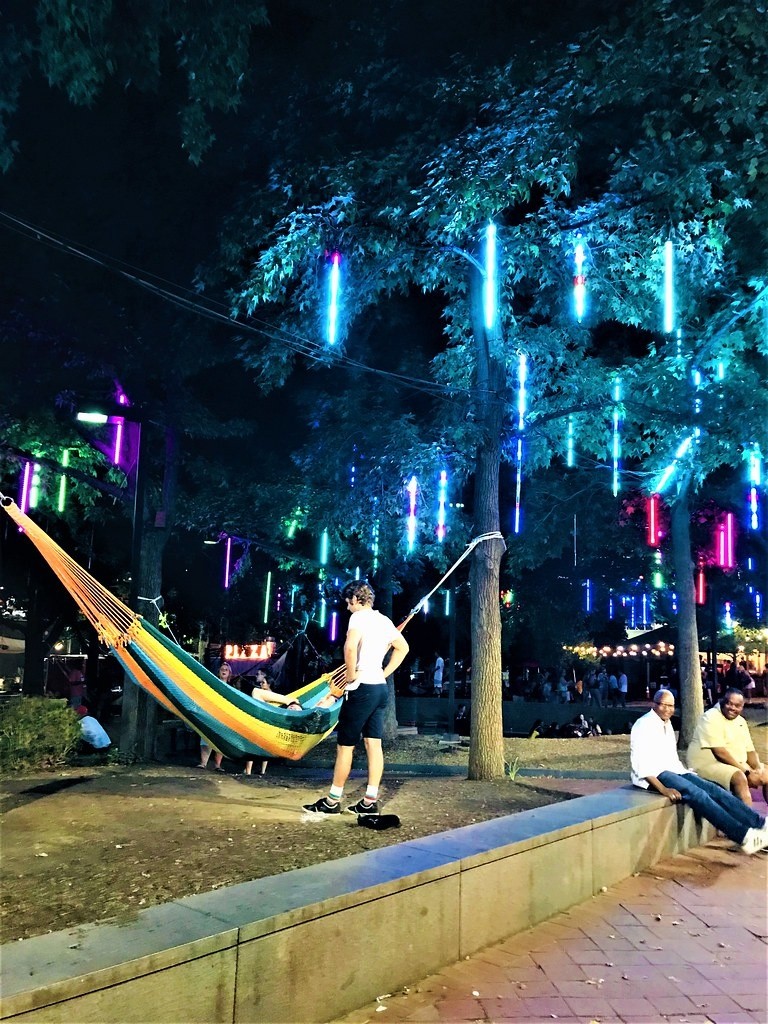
[742,828,768,855]
[301,797,341,815]
[344,798,378,814]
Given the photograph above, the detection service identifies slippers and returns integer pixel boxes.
[213,768,226,772]
[197,765,206,769]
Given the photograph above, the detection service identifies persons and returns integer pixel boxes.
[302,580,410,815]
[196,662,233,771]
[74,706,112,764]
[430,651,444,697]
[582,667,628,709]
[68,658,87,707]
[503,669,573,704]
[699,654,768,703]
[629,689,768,855]
[252,687,335,711]
[243,668,276,775]
[686,689,768,808]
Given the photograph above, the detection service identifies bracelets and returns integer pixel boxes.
[745,770,750,776]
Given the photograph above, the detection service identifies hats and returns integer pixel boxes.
[75,706,88,716]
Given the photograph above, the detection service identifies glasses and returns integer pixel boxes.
[655,701,674,709]
